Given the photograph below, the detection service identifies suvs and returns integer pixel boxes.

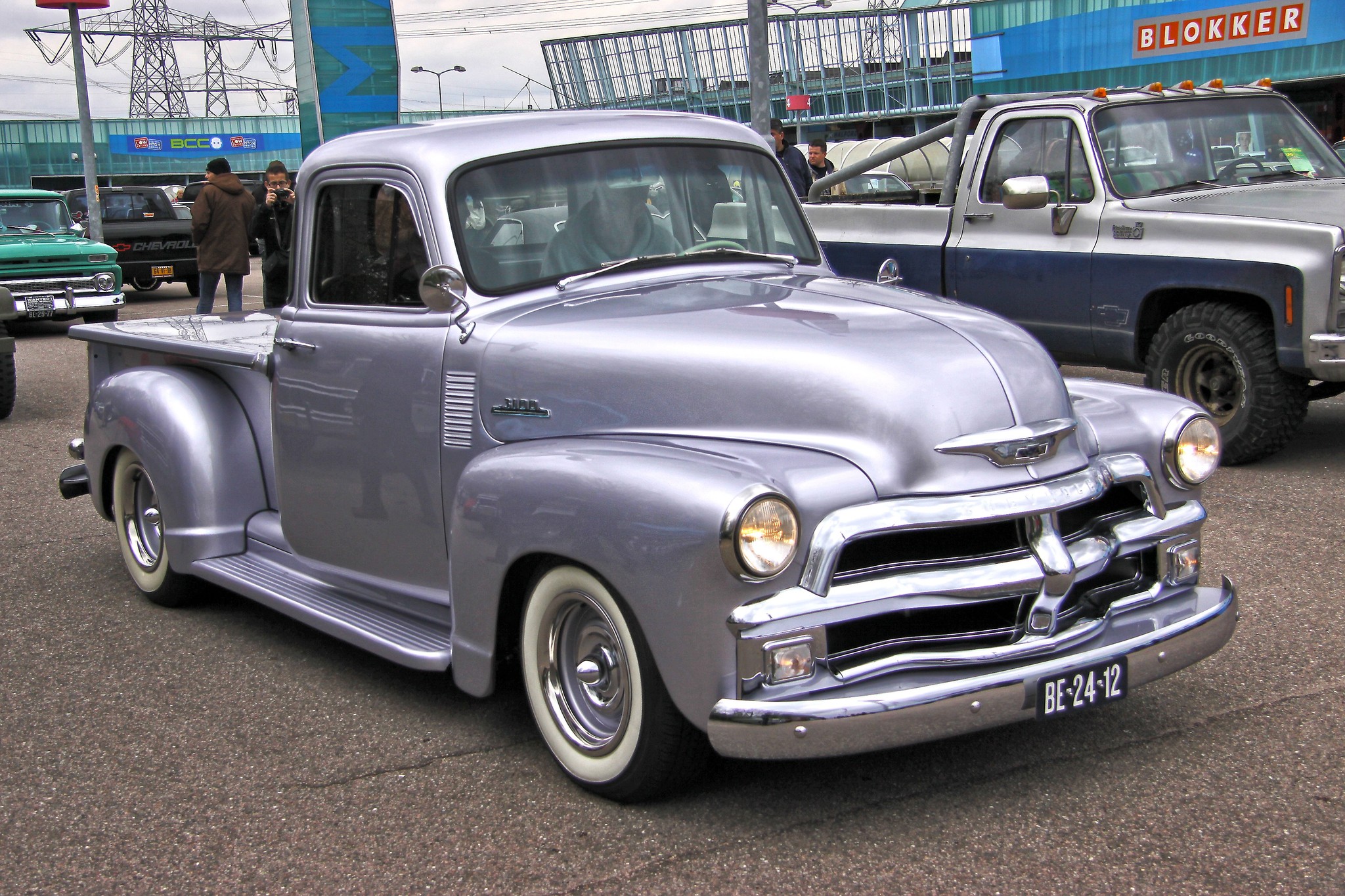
[59,185,200,297]
[0,188,126,337]
[0,286,17,419]
[181,179,265,257]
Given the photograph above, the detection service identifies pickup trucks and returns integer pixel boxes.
[707,79,1345,466]
[57,108,1238,808]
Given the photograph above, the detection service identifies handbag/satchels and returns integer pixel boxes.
[262,250,289,278]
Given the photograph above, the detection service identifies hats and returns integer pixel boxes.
[206,158,231,174]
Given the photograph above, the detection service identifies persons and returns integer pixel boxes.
[540,156,682,278]
[687,161,733,235]
[770,117,814,198]
[171,189,184,202]
[1268,139,1287,161]
[190,157,256,314]
[806,139,847,196]
[249,160,296,308]
[1238,133,1251,152]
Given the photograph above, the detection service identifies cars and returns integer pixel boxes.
[484,202,706,247]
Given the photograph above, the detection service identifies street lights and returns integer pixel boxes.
[411,66,467,119]
[767,0,832,144]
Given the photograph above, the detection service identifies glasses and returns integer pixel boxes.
[267,179,287,188]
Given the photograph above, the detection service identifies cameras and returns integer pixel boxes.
[271,188,290,200]
